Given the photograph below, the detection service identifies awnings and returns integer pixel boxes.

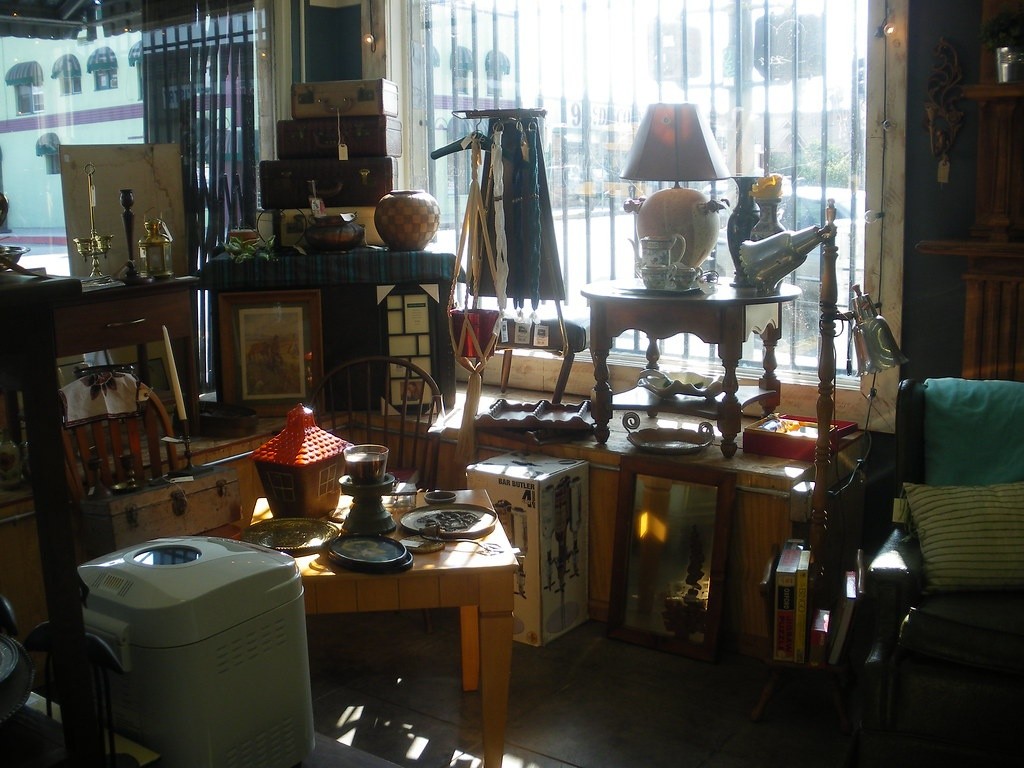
[486,50,509,74]
[450,47,472,72]
[85,47,117,72]
[128,42,142,67]
[36,133,61,156]
[433,48,440,67]
[50,54,81,78]
[4,61,43,87]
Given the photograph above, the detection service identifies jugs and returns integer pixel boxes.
[628,233,703,290]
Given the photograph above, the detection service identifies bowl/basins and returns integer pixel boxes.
[0,246,30,272]
[424,491,457,504]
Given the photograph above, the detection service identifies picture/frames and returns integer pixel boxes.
[218,289,326,418]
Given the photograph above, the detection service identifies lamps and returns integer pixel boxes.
[620,101,731,269]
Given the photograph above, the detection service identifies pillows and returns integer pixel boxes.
[900,481,1024,595]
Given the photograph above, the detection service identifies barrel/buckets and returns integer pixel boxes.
[453,309,499,359]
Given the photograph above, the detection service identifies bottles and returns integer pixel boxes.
[304,215,364,254]
[374,189,442,252]
[728,176,760,288]
[0,427,24,491]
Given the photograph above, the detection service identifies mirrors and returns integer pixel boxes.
[604,454,738,667]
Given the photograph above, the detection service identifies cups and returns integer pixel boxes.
[343,444,389,484]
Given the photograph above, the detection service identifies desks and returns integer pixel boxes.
[7,274,201,460]
[0,272,110,768]
[248,488,519,768]
[581,276,804,459]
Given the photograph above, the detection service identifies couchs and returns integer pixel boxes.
[868,377,1024,757]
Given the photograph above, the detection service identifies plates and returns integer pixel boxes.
[240,517,339,550]
[329,533,414,573]
[619,279,702,295]
[626,428,714,453]
[401,503,499,537]
[400,535,446,552]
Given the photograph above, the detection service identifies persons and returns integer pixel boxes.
[401,382,421,401]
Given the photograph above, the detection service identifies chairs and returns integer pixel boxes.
[57,371,241,543]
[309,356,443,494]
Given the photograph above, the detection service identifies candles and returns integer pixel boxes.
[342,442,391,487]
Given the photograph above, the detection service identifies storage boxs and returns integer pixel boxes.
[772,538,812,665]
[276,115,404,159]
[200,248,460,417]
[79,465,243,557]
[742,413,859,462]
[259,158,399,210]
[465,452,591,649]
[290,78,400,118]
[808,609,833,669]
